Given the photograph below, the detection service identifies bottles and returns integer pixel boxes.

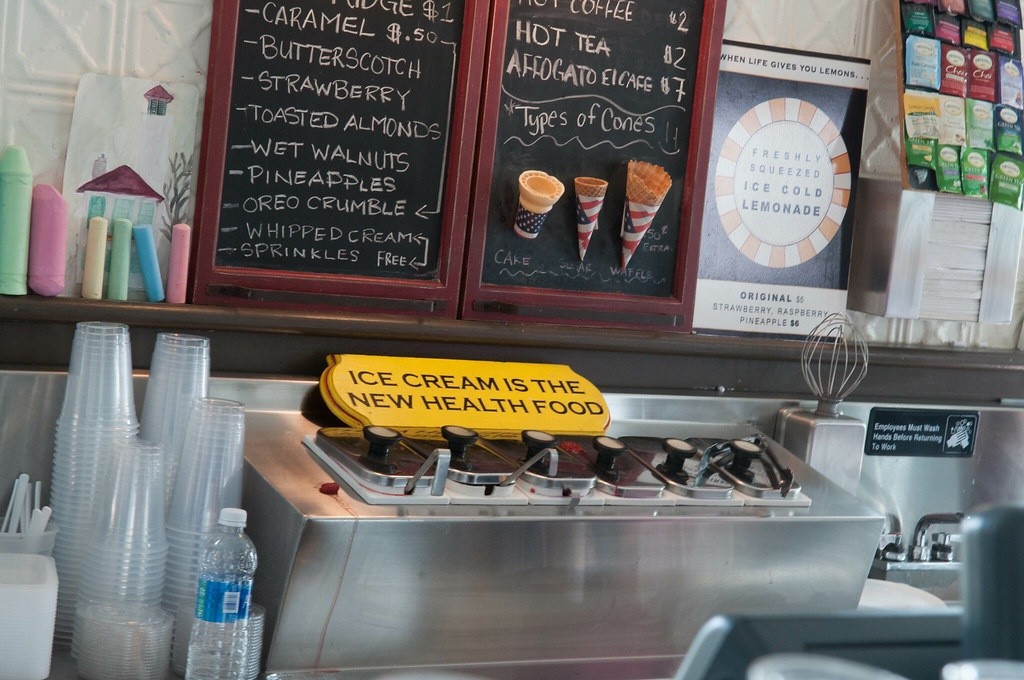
[182,507,256,680]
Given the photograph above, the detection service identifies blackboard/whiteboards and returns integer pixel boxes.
[216,0,466,287]
[482,0,703,298]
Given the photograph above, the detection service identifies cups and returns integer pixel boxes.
[0,318,244,680]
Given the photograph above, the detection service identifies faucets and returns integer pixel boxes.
[907,512,975,563]
